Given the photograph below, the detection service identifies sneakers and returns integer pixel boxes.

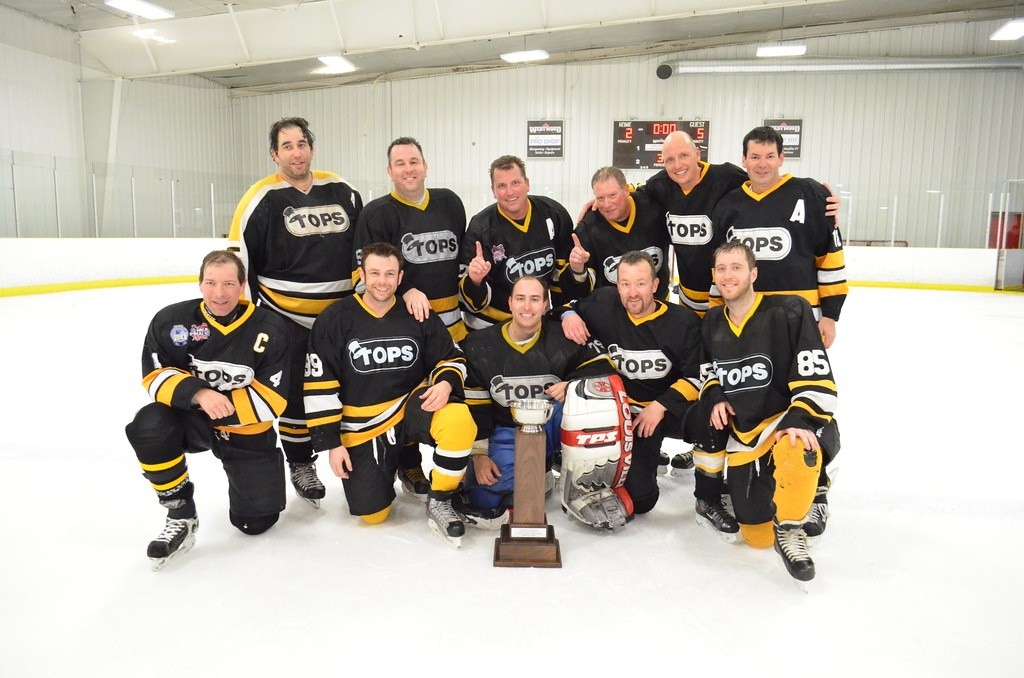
[397,467,430,504]
[694,469,739,543]
[657,448,695,476]
[800,475,831,547]
[287,454,326,509]
[424,468,467,549]
[146,482,199,574]
[772,515,816,595]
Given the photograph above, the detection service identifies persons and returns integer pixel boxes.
[570,166,671,466]
[704,126,849,536]
[546,252,742,532]
[304,243,478,538]
[692,241,842,581]
[226,117,364,499]
[125,250,292,558]
[350,136,466,496]
[454,276,634,532]
[578,126,839,469]
[457,154,573,467]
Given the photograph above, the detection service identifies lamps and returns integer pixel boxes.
[500,34,550,65]
[988,9,1024,40]
[755,7,808,60]
